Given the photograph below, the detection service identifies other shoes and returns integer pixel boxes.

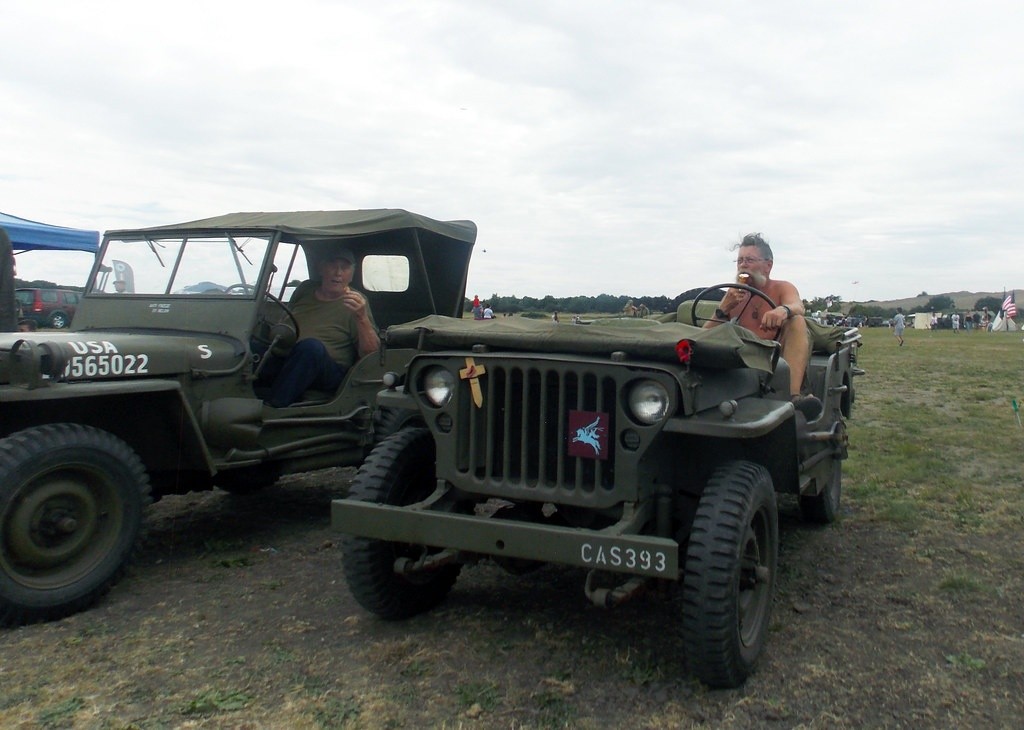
[791,394,823,421]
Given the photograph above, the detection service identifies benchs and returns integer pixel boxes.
[657,300,846,422]
[259,299,288,325]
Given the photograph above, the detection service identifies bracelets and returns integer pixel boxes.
[783,306,791,317]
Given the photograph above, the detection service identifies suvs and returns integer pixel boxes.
[331,284,868,690]
[12,288,82,330]
[0,210,480,621]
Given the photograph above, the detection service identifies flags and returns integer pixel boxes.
[1002,293,1017,319]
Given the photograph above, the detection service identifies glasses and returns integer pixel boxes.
[733,255,769,264]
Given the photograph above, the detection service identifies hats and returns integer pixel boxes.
[325,247,354,266]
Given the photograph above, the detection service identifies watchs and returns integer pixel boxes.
[715,309,729,318]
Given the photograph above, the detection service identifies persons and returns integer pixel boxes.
[113,280,128,293]
[862,315,869,327]
[573,315,579,325]
[552,312,559,322]
[18,320,36,332]
[471,295,484,320]
[965,310,987,331]
[951,311,960,333]
[250,245,381,407]
[833,317,848,327]
[892,307,904,346]
[930,313,938,332]
[701,233,823,422]
[484,304,495,320]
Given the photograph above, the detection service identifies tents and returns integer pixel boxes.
[0,211,99,288]
